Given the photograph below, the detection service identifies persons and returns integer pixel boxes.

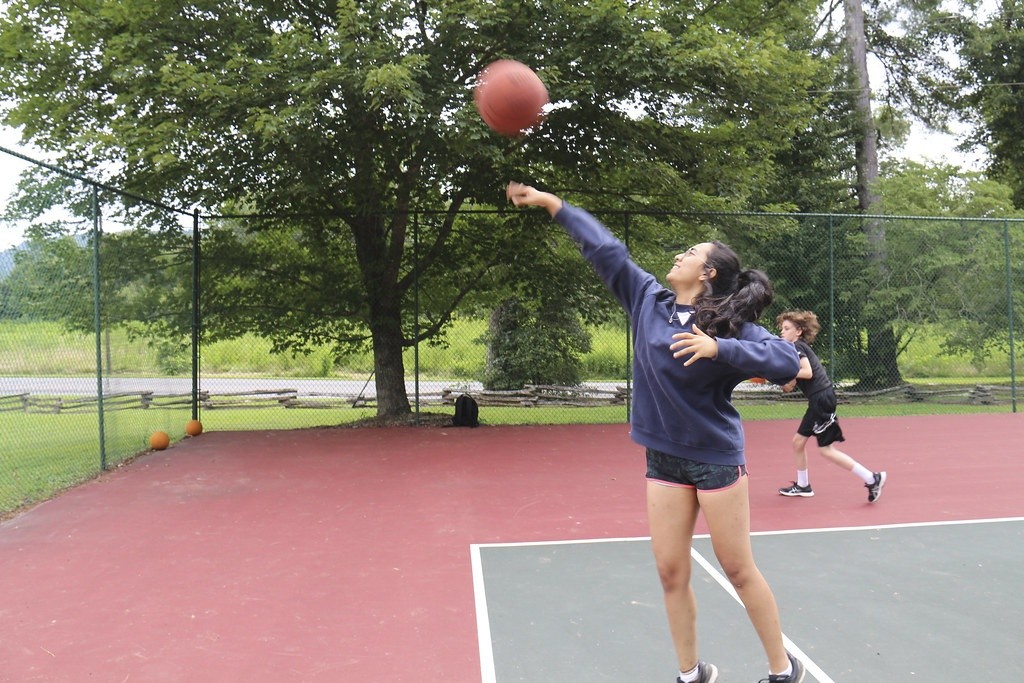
[506,180,805,682]
[776,312,886,502]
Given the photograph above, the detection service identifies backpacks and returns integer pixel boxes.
[450,392,480,428]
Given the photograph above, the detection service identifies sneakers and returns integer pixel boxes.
[863,470,888,502]
[778,481,815,497]
[674,660,718,683]
[769,652,805,683]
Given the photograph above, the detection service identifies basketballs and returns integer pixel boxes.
[186,420,202,435]
[476,59,546,135]
[149,431,169,453]
